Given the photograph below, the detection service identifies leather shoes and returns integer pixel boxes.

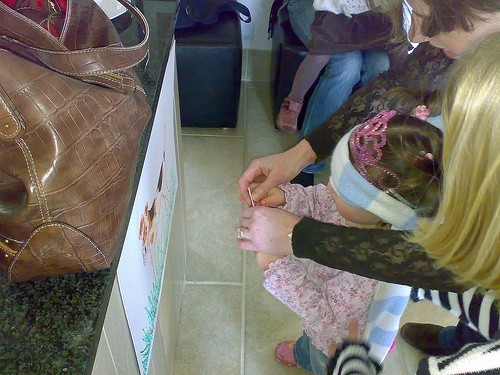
[400,322,444,357]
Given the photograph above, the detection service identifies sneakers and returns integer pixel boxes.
[276,95,303,134]
[274,341,300,368]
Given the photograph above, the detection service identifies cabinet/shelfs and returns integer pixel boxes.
[0,0,180,375]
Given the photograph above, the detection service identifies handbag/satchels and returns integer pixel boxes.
[0,0,153,282]
[173,0,251,36]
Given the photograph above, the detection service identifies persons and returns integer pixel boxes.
[325,30,500,375]
[276,0,409,135]
[235,0,500,357]
[240,113,443,375]
[287,0,391,187]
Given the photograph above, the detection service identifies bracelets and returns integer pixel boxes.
[288,229,294,249]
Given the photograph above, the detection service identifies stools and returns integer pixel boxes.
[177,11,242,128]
[269,2,328,130]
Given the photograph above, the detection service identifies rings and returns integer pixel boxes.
[237,227,246,239]
